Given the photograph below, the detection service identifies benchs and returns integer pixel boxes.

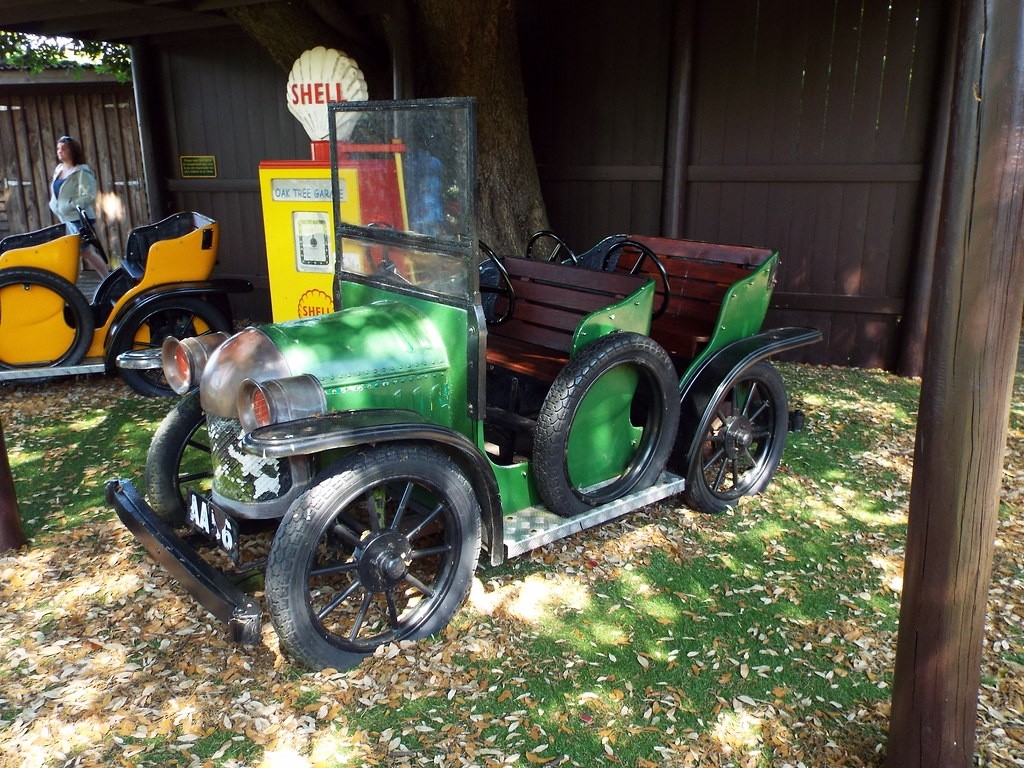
[121,211,217,295]
[478,255,655,386]
[561,234,781,368]
[0,221,80,282]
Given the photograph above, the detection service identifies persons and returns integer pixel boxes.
[49,136,111,287]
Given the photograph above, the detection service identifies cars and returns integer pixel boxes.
[101,96,822,675]
[0,213,262,403]
